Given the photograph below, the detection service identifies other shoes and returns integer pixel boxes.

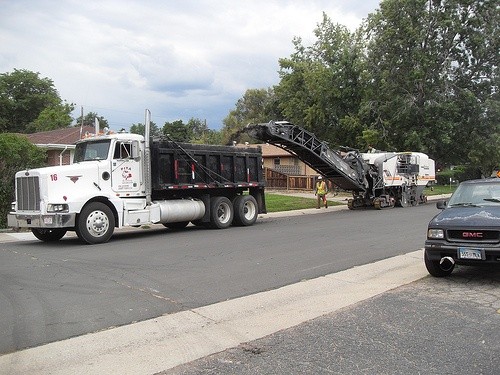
[316,206,320,209]
[324,204,327,208]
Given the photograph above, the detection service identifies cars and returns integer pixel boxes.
[423,176,500,276]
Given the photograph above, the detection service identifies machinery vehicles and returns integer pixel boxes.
[246,119,436,210]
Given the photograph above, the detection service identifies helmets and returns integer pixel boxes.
[318,176,323,179]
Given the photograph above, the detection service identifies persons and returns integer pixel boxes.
[314,176,329,209]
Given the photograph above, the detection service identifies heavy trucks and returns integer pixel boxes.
[7,107,268,246]
